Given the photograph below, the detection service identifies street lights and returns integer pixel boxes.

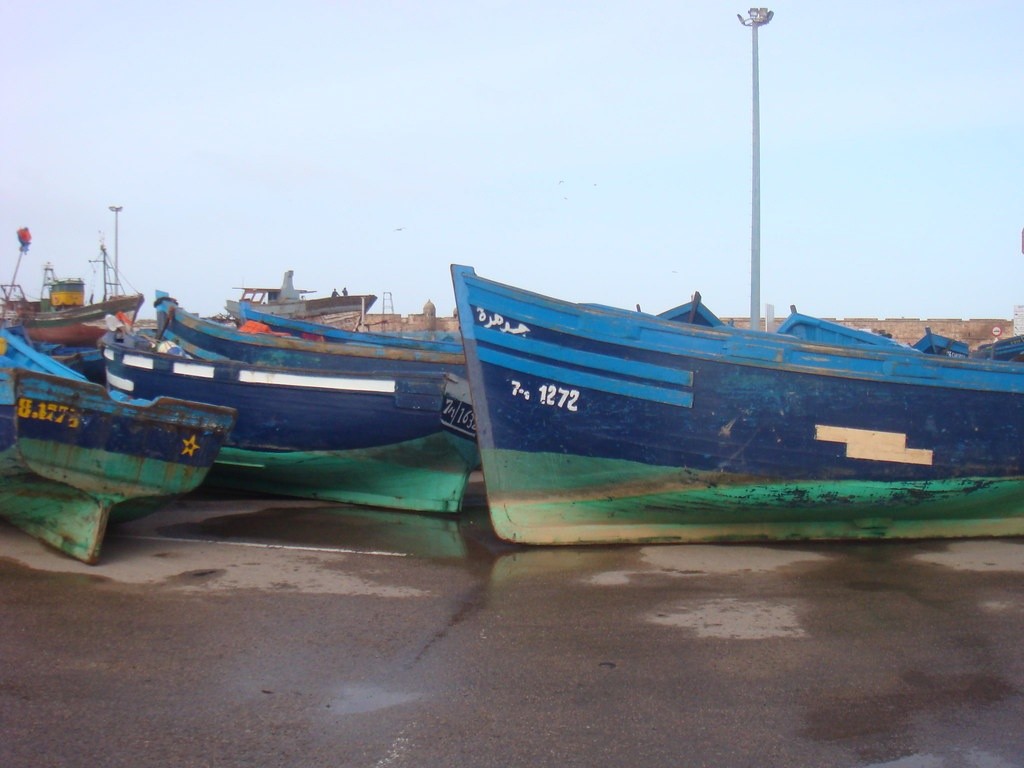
[108,205,123,297]
[737,6,774,331]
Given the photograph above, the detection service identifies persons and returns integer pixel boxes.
[342,288,348,296]
[332,289,340,298]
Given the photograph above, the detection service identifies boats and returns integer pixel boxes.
[448,263,1024,548]
[971,333,1024,362]
[908,325,969,358]
[225,283,379,333]
[0,327,239,568]
[6,289,482,515]
[635,289,725,326]
[776,304,925,354]
[0,232,146,346]
